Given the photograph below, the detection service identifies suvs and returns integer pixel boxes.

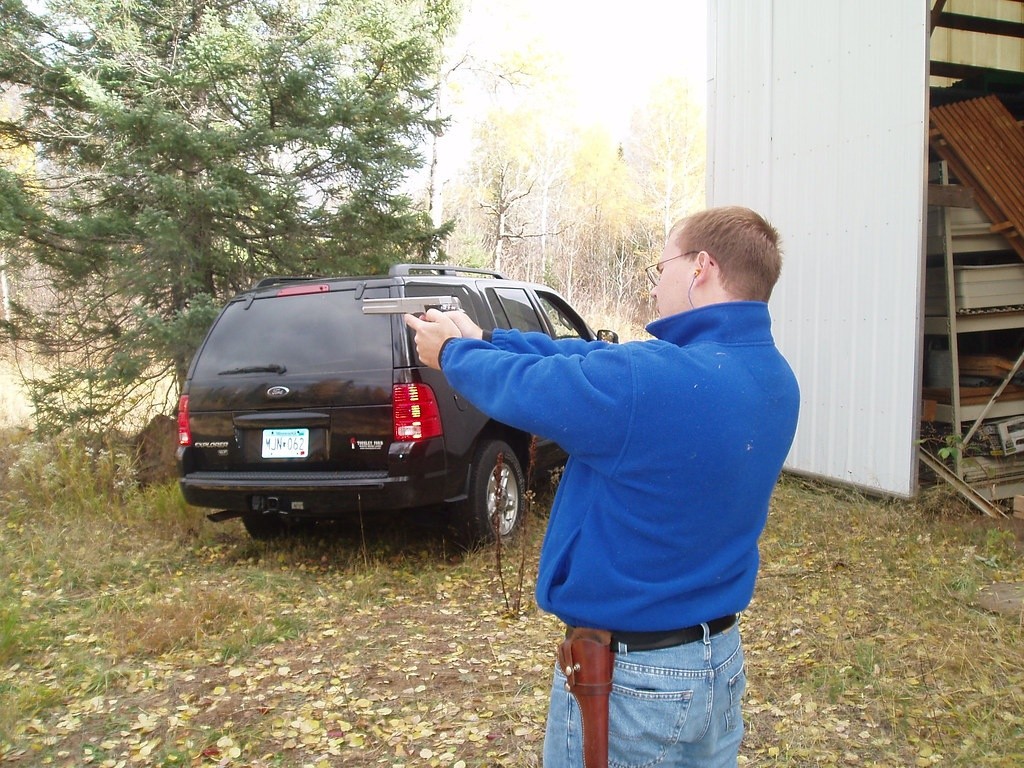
[172,264,618,546]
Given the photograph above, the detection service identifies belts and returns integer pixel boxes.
[565,611,736,653]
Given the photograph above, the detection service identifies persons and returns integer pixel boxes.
[406,206,801,768]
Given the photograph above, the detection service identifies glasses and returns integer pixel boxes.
[644,251,715,286]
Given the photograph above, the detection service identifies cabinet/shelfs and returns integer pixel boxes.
[926,160,1024,502]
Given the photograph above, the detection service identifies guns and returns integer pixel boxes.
[361,295,464,319]
